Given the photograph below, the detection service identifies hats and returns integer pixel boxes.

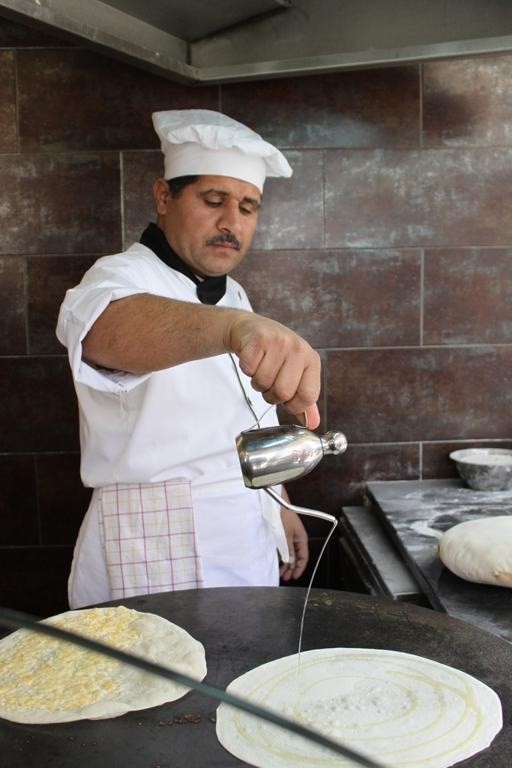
[151,108,293,194]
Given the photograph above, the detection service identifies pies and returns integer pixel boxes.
[0,606,207,724]
[216,646,502,767]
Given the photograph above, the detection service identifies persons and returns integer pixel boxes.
[52,102,325,618]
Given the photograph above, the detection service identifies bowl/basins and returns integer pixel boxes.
[447,444,512,493]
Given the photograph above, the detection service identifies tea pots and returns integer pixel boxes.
[235,399,349,527]
